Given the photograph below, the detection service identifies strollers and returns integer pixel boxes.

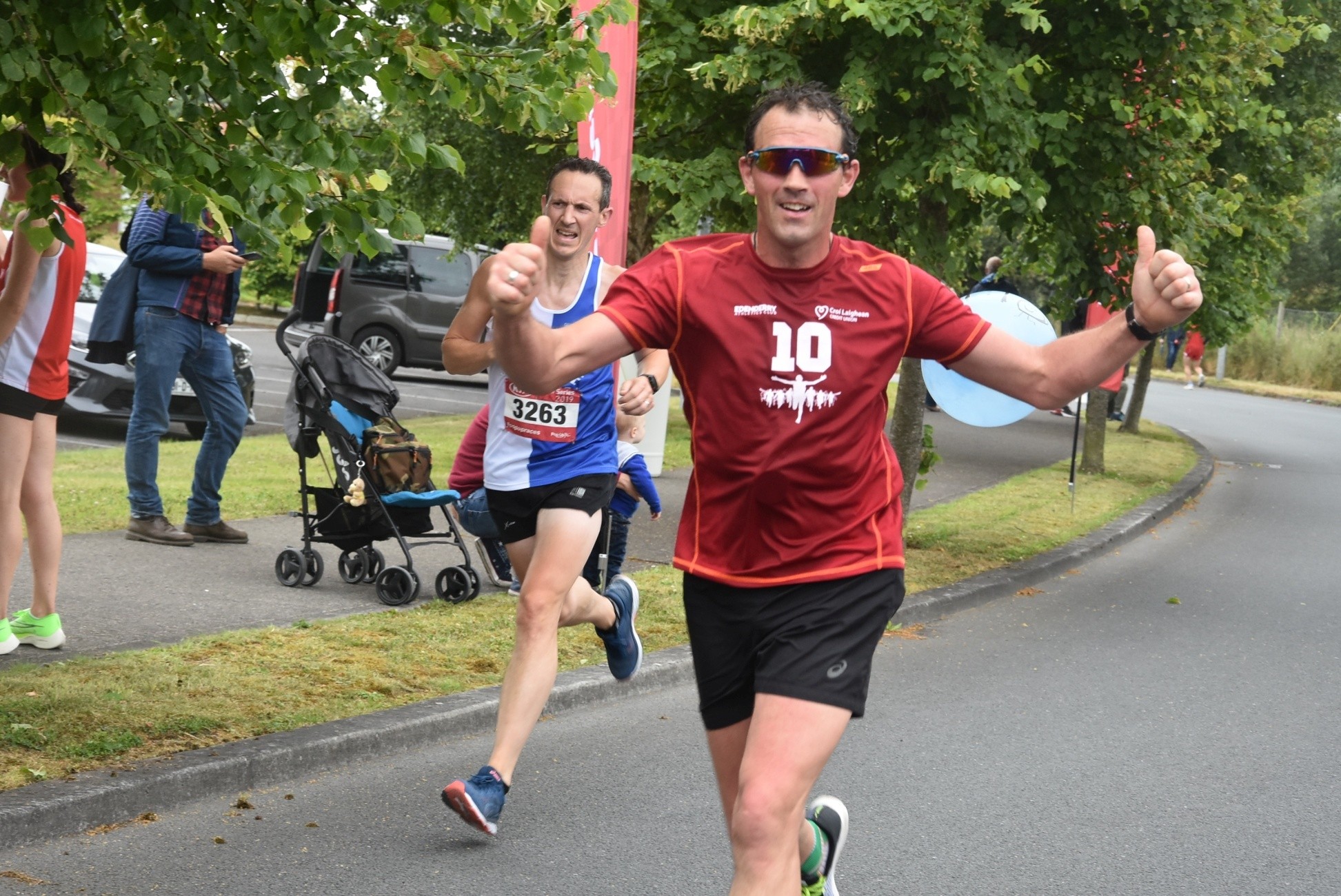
[275,310,481,607]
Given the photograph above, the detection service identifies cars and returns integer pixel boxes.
[2,230,257,439]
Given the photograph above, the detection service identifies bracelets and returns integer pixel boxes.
[635,374,659,395]
[1125,302,1162,344]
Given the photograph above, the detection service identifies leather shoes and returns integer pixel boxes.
[125,515,193,545]
[184,520,248,542]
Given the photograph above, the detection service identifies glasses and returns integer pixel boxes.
[748,147,849,176]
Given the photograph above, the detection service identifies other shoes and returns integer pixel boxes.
[1198,374,1205,386]
[1051,405,1078,419]
[1184,385,1193,389]
[1110,410,1127,423]
[924,402,940,412]
[508,581,522,596]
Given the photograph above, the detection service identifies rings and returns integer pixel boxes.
[507,270,520,284]
[643,401,650,406]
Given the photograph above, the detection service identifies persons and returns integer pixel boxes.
[970,256,1021,295]
[0,132,88,657]
[125,188,250,547]
[88,391,88,394]
[1165,325,1186,371]
[1184,320,1206,391]
[583,411,663,592]
[438,81,1207,896]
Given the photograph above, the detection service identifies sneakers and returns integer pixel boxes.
[475,536,517,586]
[10,609,66,648]
[0,618,20,654]
[441,766,512,835]
[800,795,849,896]
[593,575,641,680]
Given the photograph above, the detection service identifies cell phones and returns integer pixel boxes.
[238,251,263,261]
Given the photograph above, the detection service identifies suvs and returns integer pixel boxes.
[281,224,502,380]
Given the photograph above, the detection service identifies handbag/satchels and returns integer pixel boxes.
[359,415,434,495]
